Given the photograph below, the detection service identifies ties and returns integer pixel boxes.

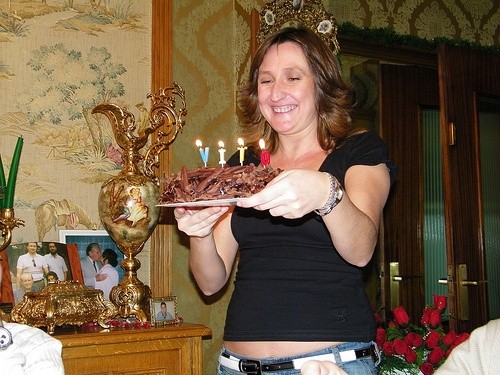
[93,261,97,273]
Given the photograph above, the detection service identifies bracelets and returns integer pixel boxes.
[318,173,335,211]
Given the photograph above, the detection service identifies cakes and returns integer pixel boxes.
[158,165,286,204]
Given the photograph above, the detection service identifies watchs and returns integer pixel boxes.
[315,181,343,216]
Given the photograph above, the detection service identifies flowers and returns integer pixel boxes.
[375,299,461,375]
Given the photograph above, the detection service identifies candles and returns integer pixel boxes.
[217,141,226,164]
[195,139,210,163]
[0,134,24,213]
[237,138,246,160]
[258,140,271,165]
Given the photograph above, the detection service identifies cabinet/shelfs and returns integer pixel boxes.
[52,322,212,374]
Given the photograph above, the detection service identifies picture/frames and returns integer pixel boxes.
[59,228,133,300]
[6,242,86,308]
[150,296,180,326]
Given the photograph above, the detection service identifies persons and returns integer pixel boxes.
[157,302,172,319]
[81,243,118,298]
[10,243,68,304]
[301,319,500,375]
[174,27,390,375]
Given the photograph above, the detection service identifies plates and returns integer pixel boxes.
[155,197,246,207]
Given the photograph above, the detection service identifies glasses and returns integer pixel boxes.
[32,259,36,267]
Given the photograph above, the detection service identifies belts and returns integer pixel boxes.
[217,345,372,375]
[33,279,44,283]
